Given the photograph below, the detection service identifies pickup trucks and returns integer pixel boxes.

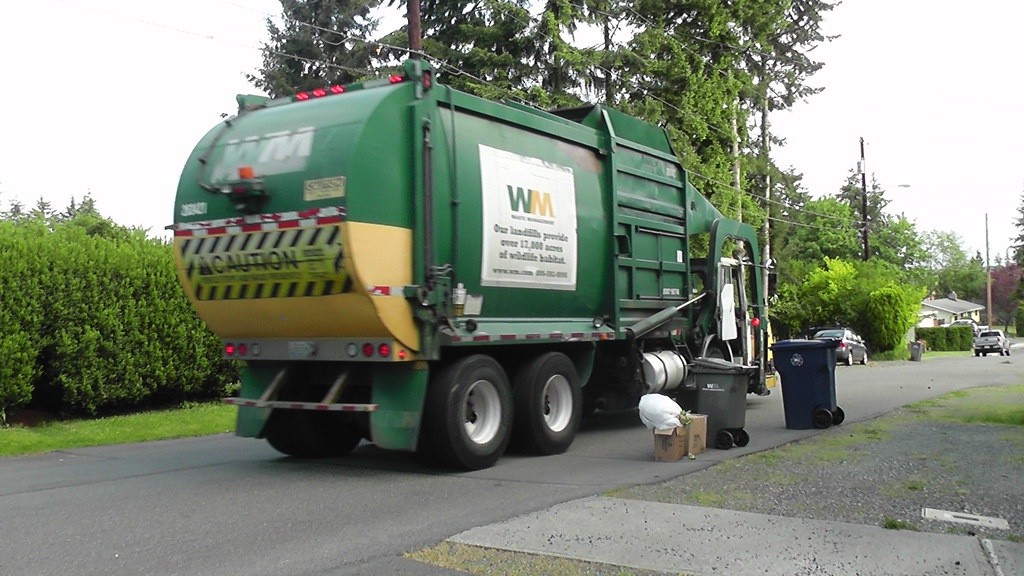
[973,329,1010,357]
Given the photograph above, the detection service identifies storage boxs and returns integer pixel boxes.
[652,427,686,462]
[685,414,708,456]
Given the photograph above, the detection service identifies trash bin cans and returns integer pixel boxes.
[769,339,845,430]
[689,356,760,451]
[910,342,922,362]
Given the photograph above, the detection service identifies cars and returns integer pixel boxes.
[814,327,869,366]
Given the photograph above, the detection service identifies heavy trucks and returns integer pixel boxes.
[161,60,779,475]
